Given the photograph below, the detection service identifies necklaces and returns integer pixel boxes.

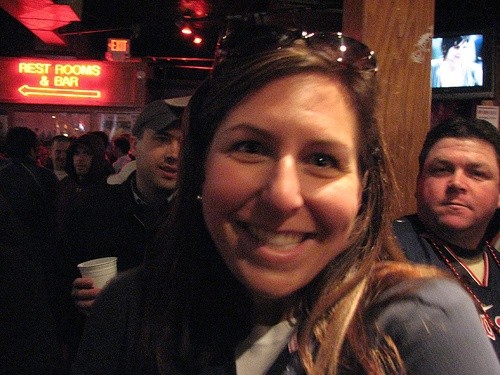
[425,229,500,337]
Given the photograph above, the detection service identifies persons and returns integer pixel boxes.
[432,35,476,87]
[0,102,185,375]
[380,118,500,361]
[72,26,500,375]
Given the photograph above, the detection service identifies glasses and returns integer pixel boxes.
[214,26,378,77]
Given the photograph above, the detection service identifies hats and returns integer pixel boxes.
[131,102,186,133]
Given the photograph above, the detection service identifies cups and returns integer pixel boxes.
[77,257,117,291]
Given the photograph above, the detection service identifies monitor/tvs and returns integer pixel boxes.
[430,28,495,100]
